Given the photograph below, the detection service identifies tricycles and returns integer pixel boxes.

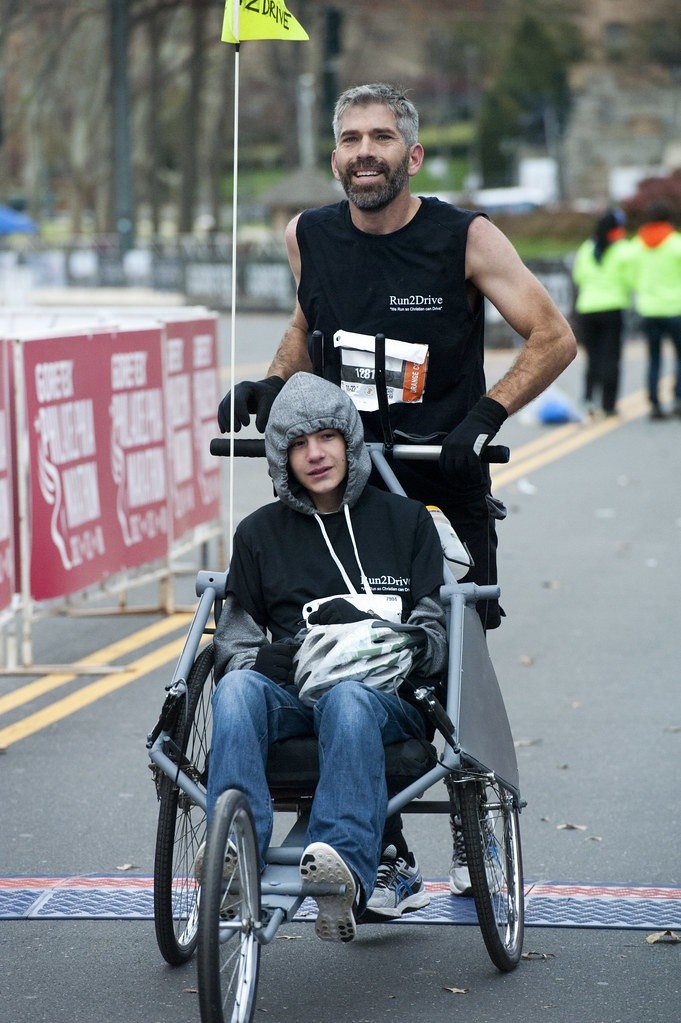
[147,328,526,1023]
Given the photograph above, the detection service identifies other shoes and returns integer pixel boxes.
[299,842,358,944]
[193,840,243,922]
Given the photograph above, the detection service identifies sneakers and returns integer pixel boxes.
[449,813,506,898]
[363,852,431,920]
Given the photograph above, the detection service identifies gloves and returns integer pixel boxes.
[216,374,284,434]
[307,598,374,626]
[253,642,300,685]
[439,396,508,487]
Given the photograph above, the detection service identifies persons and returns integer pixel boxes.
[630,196,681,419]
[218,82,578,920]
[193,370,448,944]
[572,203,635,418]
